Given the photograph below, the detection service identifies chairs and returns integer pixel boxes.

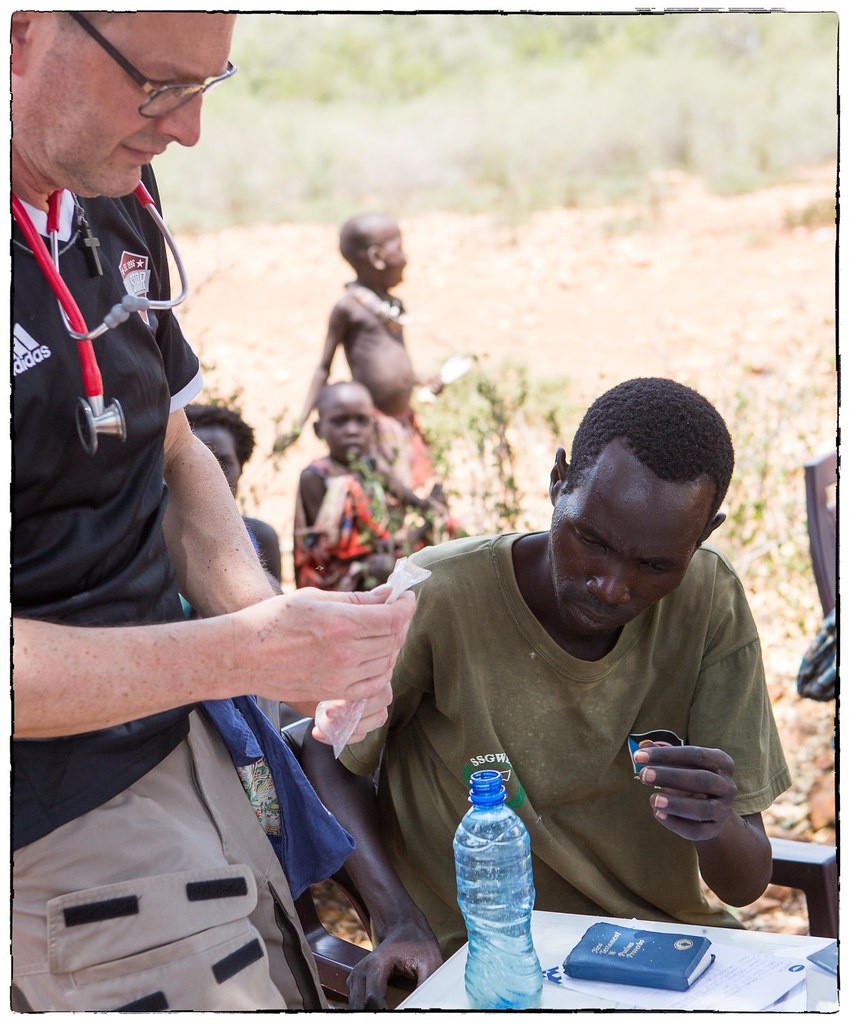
[281,718,836,1009]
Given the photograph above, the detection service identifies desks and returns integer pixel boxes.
[394,909,838,1011]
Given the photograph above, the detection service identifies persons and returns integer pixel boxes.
[10,8,469,1010]
[300,378,793,1010]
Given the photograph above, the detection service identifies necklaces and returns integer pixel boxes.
[10,188,104,275]
[345,282,410,327]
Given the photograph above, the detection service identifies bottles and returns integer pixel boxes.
[454,770,544,1009]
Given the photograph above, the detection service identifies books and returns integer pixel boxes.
[562,922,715,991]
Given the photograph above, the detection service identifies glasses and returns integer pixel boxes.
[68,12,238,118]
